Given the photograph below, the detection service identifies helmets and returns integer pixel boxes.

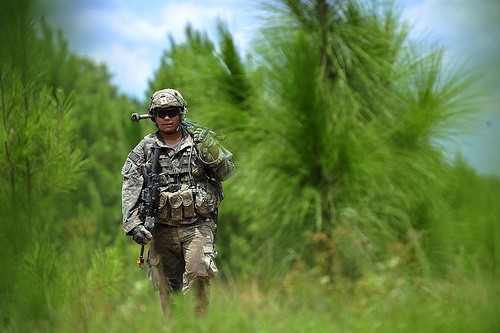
[148,88,186,114]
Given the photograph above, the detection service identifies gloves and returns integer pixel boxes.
[193,128,214,147]
[132,227,153,246]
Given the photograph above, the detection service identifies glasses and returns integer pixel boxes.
[155,108,180,118]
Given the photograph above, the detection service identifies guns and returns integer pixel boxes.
[136,146,163,265]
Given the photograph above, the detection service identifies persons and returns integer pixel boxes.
[122,89,235,323]
